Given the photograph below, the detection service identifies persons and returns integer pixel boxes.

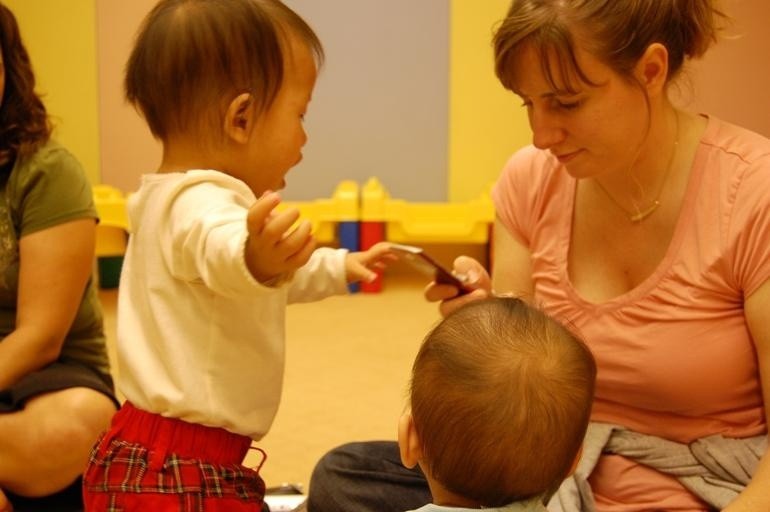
[0,0,123,511]
[80,0,405,511]
[392,293,598,512]
[305,0,770,512]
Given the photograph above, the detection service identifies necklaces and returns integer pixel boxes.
[586,107,687,229]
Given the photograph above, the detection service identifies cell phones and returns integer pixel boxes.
[389,245,468,295]
